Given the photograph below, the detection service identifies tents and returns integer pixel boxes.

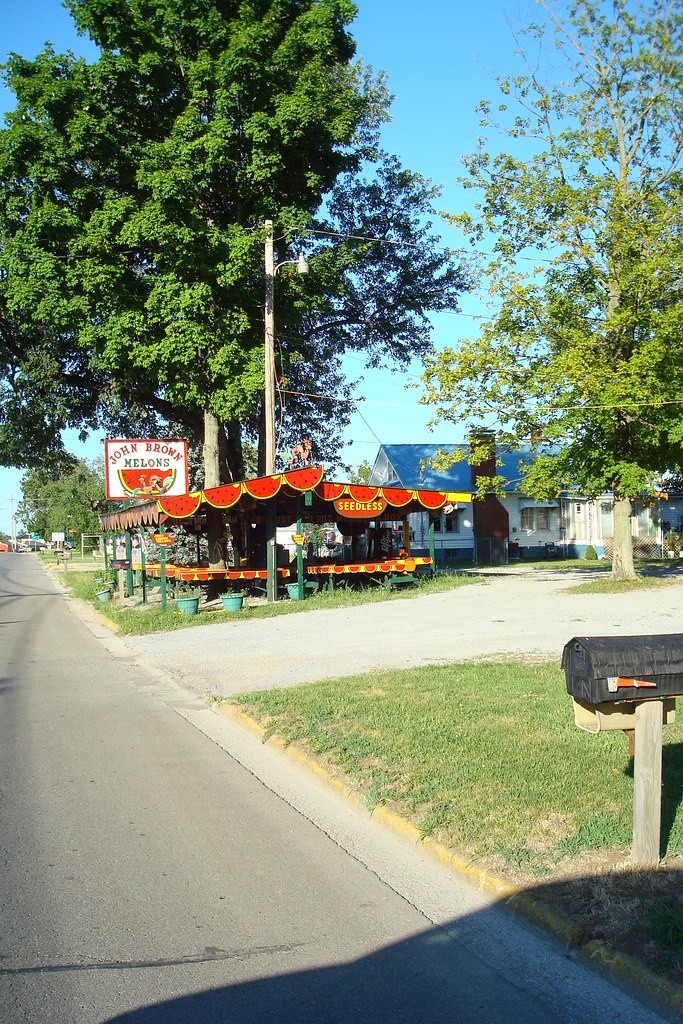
[98,464,474,616]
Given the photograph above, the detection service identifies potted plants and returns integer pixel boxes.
[94,569,104,583]
[665,529,679,559]
[103,574,115,592]
[173,588,203,615]
[218,589,248,611]
[678,532,683,557]
[94,586,111,602]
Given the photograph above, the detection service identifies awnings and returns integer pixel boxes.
[457,502,467,513]
[518,497,559,513]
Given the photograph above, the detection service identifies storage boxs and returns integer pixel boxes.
[285,579,307,600]
